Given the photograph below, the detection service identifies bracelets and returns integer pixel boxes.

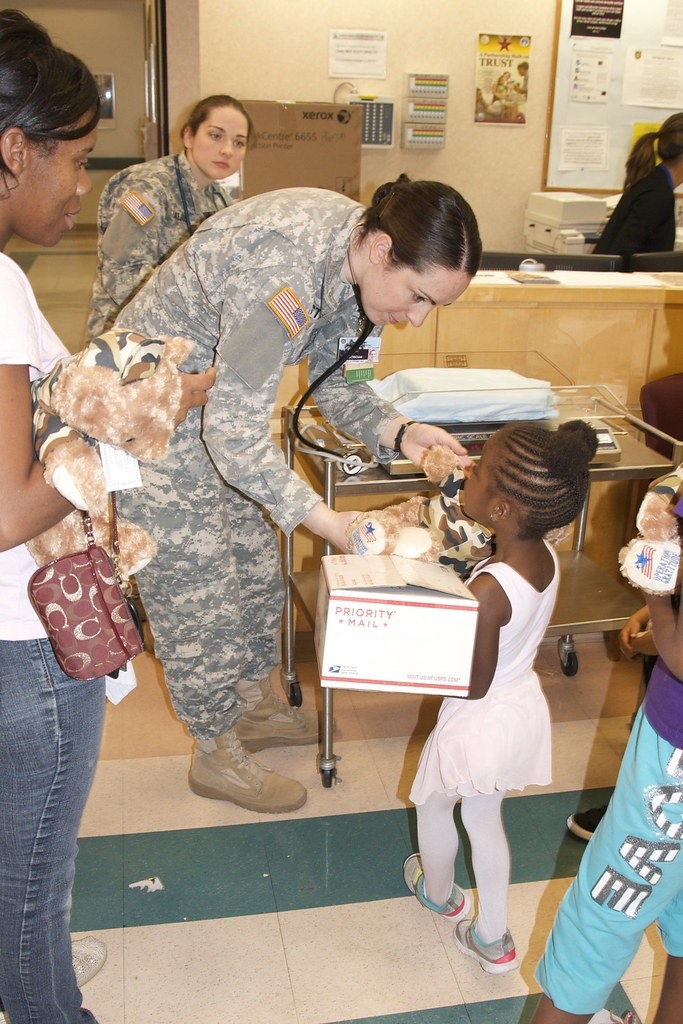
[393,421,417,452]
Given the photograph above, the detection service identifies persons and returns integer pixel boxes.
[403,420,598,975]
[592,112,683,254]
[113,172,484,813]
[476,62,529,123]
[529,464,682,1024]
[0,6,216,1023]
[86,94,253,341]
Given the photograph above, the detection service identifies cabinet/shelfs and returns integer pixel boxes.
[283,393,683,789]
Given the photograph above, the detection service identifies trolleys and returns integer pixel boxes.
[280,395,683,789]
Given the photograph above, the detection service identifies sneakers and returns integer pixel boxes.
[188,727,307,814]
[233,677,336,753]
[453,918,520,975]
[403,853,471,921]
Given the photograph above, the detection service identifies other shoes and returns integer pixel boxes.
[0,936,107,1024]
[566,805,608,840]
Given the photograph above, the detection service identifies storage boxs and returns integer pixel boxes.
[314,553,482,699]
[240,99,361,203]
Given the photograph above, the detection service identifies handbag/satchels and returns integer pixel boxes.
[27,491,146,682]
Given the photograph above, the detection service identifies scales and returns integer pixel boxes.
[321,386,629,477]
